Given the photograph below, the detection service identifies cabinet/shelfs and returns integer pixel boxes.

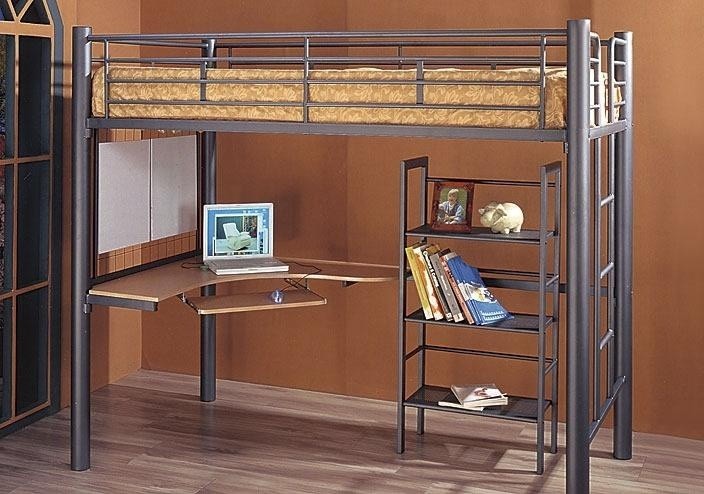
[397,156,561,475]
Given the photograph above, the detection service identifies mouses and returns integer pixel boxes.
[269,288,284,303]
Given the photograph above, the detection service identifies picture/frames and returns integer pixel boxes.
[429,181,474,233]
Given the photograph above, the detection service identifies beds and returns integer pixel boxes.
[71,19,633,494]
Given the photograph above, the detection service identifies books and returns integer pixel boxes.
[438,392,485,412]
[451,382,508,408]
[404,242,514,326]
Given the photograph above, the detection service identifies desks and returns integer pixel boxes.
[86,255,410,316]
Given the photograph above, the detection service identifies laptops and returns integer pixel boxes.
[203,202,289,275]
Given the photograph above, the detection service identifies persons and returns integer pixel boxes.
[436,189,465,225]
